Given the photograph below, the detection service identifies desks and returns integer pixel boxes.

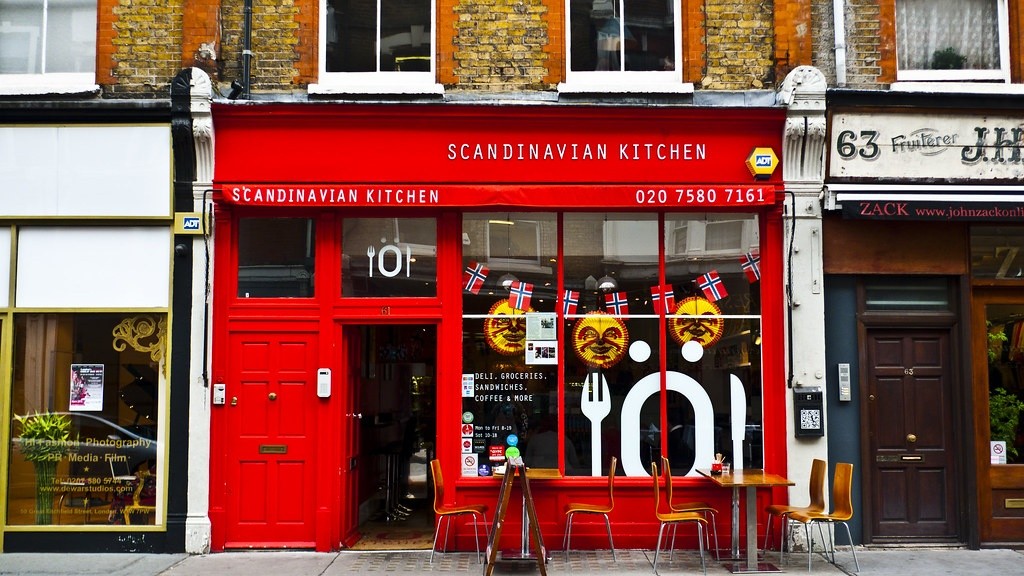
[492,468,562,560]
[695,468,795,574]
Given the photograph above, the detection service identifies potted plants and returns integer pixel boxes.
[11,408,74,525]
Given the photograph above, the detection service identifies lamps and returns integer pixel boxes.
[496,213,519,297]
[596,213,618,292]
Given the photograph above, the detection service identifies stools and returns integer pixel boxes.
[57,482,122,525]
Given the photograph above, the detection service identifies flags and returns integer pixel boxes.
[508,281,534,312]
[697,269,729,303]
[738,249,761,284]
[605,292,629,321]
[554,289,580,321]
[462,260,490,295]
[649,284,676,314]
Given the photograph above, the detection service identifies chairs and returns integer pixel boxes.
[786,462,861,573]
[661,455,721,564]
[651,462,708,576]
[763,459,830,567]
[562,456,616,555]
[430,460,485,565]
[565,457,617,564]
[434,459,490,558]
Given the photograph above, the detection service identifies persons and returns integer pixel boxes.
[600,415,626,476]
[517,416,581,470]
[527,343,555,359]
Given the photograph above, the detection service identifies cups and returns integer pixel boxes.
[722,462,730,476]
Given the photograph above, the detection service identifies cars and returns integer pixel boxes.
[8,411,157,498]
